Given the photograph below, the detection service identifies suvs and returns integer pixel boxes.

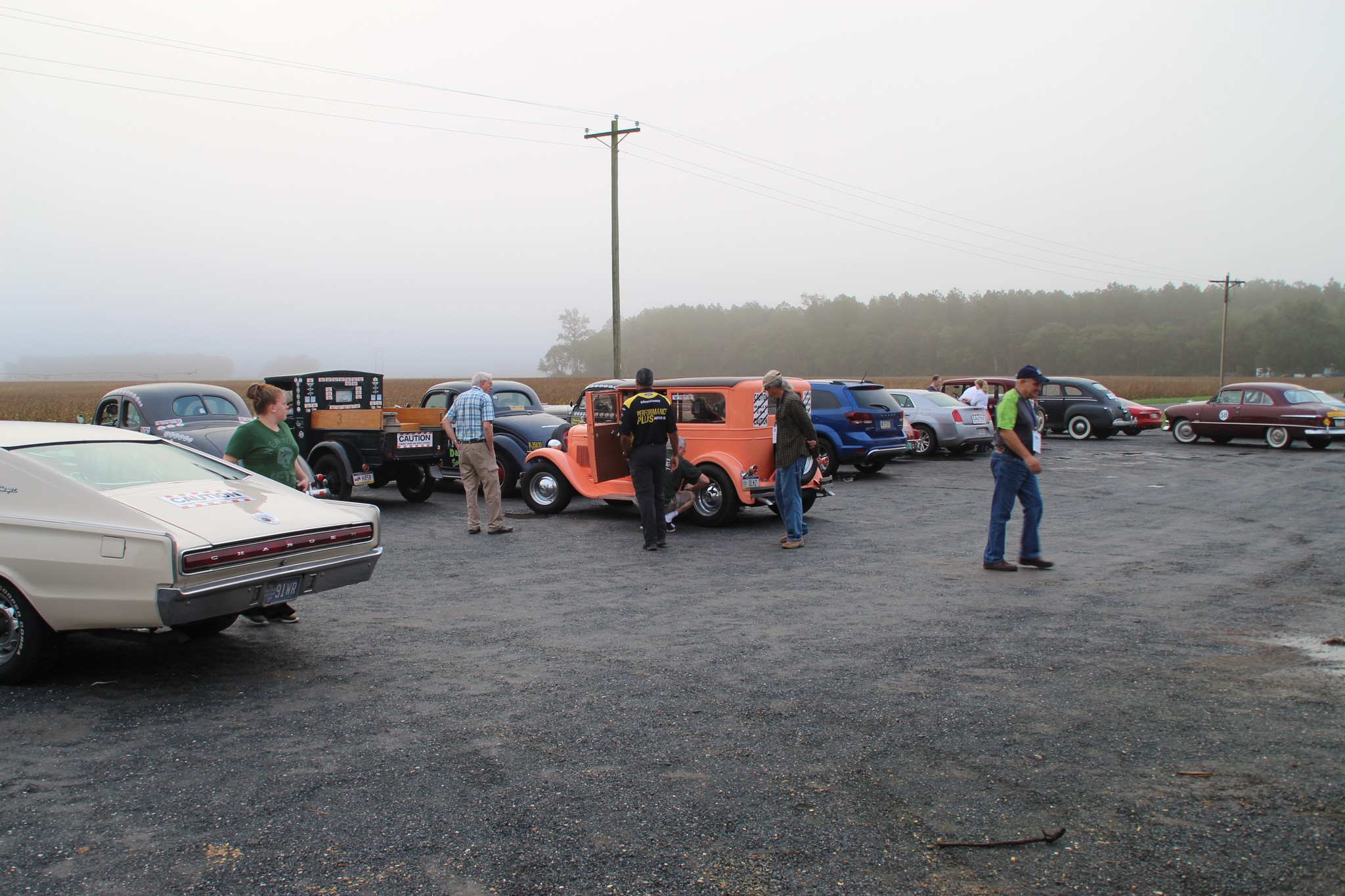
[712,378,908,477]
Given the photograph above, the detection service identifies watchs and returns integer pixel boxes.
[623,452,629,458]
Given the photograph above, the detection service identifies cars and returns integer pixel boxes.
[884,388,994,458]
[519,376,836,528]
[941,377,1051,450]
[392,380,574,497]
[75,382,331,498]
[540,394,725,424]
[1161,382,1345,450]
[1239,389,1344,408]
[1321,369,1345,378]
[1116,396,1163,436]
[1038,376,1138,441]
[0,418,385,685]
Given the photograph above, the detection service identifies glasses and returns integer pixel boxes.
[635,380,638,383]
[764,385,770,393]
[680,446,686,455]
[481,381,492,386]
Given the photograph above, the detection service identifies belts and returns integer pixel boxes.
[995,445,1022,458]
[460,439,486,444]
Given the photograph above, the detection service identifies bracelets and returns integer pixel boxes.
[452,440,458,444]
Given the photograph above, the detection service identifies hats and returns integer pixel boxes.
[1016,365,1049,382]
[761,370,782,393]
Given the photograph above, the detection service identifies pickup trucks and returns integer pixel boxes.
[263,369,449,502]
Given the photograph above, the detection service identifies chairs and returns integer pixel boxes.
[500,398,515,407]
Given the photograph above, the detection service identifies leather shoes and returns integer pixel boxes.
[983,560,1017,571]
[1019,557,1053,568]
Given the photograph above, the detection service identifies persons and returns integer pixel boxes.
[761,370,821,549]
[618,368,679,550]
[983,365,1053,571]
[223,384,309,625]
[691,397,723,422]
[441,372,513,534]
[927,375,941,392]
[632,436,709,532]
[958,379,989,453]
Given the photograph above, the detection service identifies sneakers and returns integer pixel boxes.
[782,538,804,549]
[469,527,481,534]
[780,535,788,542]
[665,520,675,532]
[243,614,269,624]
[268,613,299,622]
[640,523,643,529]
[643,543,657,550]
[657,538,666,545]
[488,525,513,534]
